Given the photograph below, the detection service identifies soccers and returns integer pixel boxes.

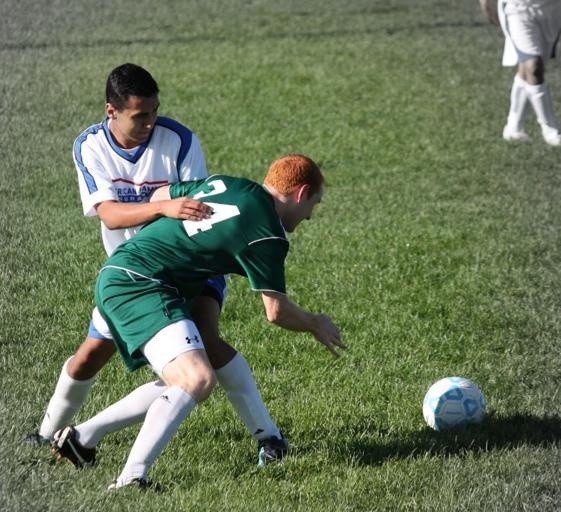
[423,377,487,433]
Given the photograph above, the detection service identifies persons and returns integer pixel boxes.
[48,153,349,492]
[24,62,308,470]
[480,0,561,148]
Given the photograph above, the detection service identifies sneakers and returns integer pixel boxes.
[24,430,52,449]
[256,428,288,468]
[106,477,163,493]
[46,422,100,469]
[541,127,561,146]
[502,125,533,141]
